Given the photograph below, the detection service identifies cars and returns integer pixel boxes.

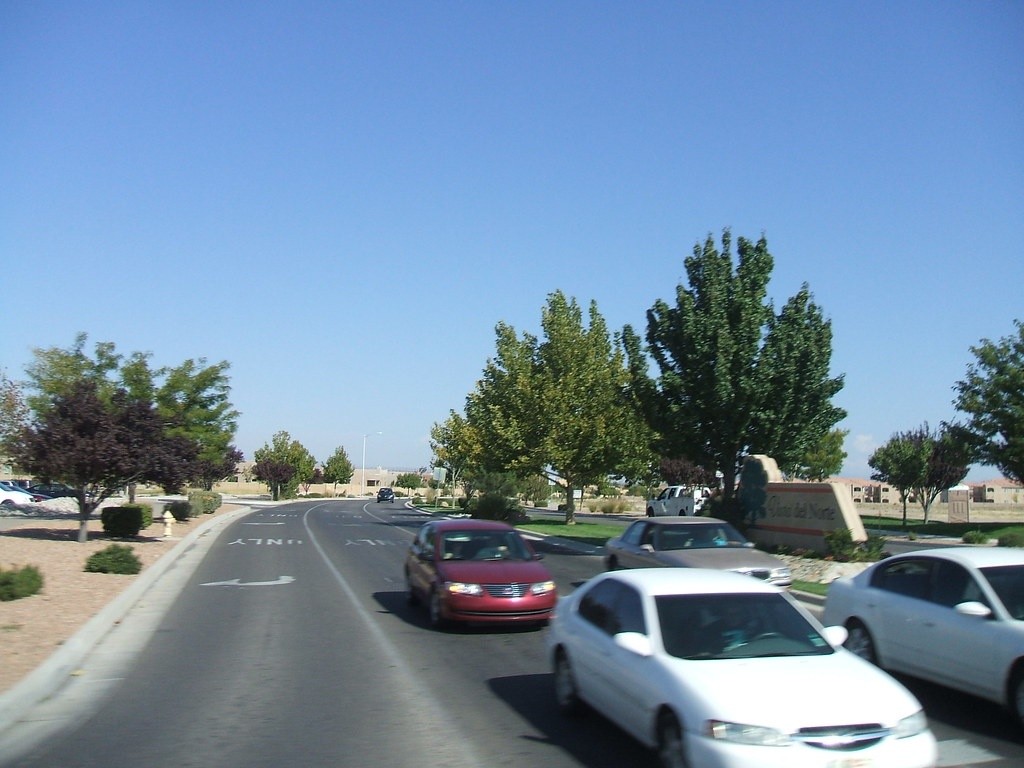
[602,515,793,595]
[544,566,942,768]
[376,488,396,503]
[403,517,559,630]
[819,546,1024,738]
[0,480,97,508]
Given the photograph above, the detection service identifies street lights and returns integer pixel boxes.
[361,431,382,496]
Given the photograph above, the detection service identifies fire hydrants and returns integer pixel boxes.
[161,510,177,538]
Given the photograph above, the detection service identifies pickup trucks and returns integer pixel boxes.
[644,485,713,517]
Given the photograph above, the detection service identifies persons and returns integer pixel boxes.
[701,597,765,652]
[692,527,742,547]
[476,537,511,558]
[449,542,467,559]
